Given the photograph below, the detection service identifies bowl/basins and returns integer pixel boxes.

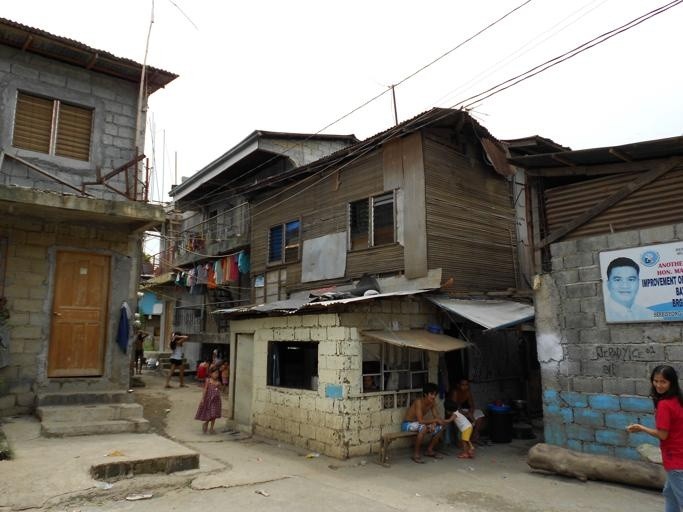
[512,400,528,407]
[428,325,441,333]
[488,403,511,412]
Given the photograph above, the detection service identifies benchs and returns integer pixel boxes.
[374,423,456,467]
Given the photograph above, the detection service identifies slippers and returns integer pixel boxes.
[410,452,444,464]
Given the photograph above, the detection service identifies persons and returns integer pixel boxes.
[164,332,189,388]
[193,366,222,433]
[134,330,148,374]
[605,257,662,321]
[625,364,683,511]
[400,381,445,463]
[219,360,228,394]
[212,344,222,363]
[196,355,209,377]
[440,399,473,459]
[452,377,484,445]
[363,375,379,393]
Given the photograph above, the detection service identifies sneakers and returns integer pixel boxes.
[456,449,475,459]
